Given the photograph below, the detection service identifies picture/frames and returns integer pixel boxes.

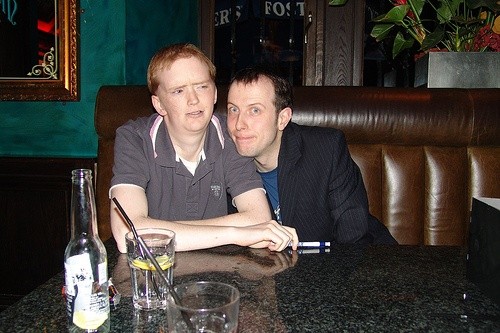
[0,0,79,103]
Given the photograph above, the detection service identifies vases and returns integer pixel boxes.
[415,52,500,88]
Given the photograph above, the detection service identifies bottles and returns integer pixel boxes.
[64,169,111,333]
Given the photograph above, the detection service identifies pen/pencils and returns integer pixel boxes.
[287,248,331,255]
[288,241,331,247]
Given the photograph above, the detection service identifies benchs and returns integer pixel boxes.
[94,84,500,246]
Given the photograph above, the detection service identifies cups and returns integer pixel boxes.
[165,281,240,333]
[134,308,176,333]
[125,227,175,310]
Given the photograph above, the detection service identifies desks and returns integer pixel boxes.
[0,235,499,332]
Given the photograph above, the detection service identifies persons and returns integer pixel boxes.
[111,249,298,300]
[107,43,299,255]
[225,65,398,247]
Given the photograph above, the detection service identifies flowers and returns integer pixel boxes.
[329,0,500,61]
